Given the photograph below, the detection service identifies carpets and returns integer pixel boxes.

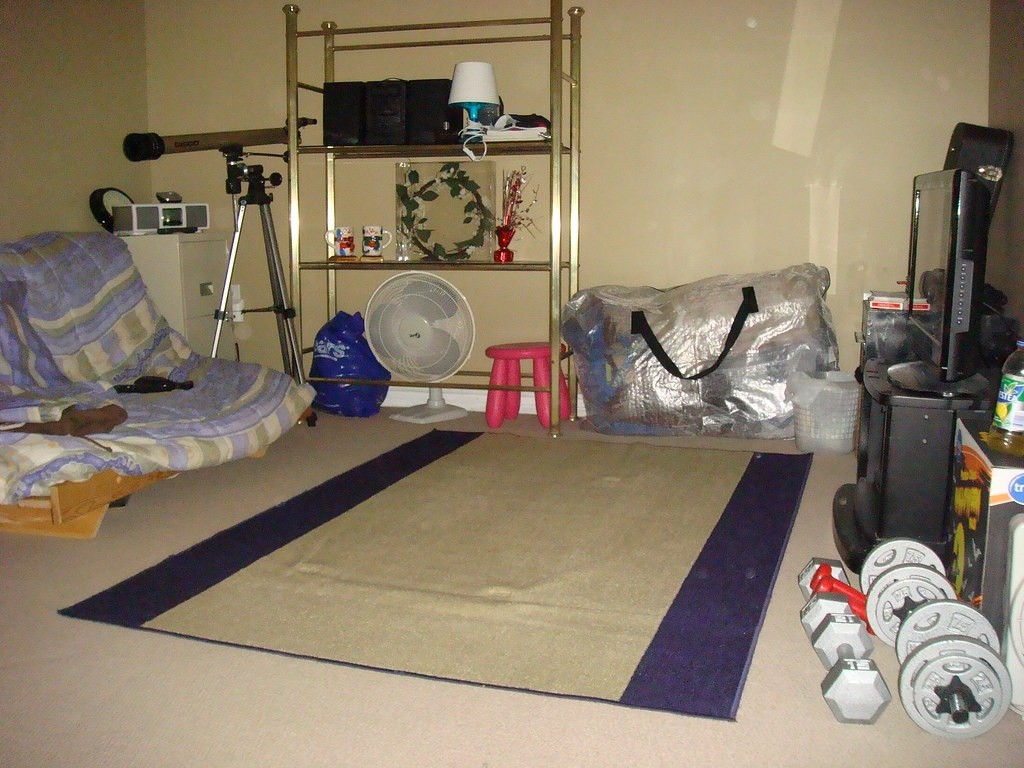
[58,429,813,723]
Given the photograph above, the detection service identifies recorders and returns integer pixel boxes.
[111,204,211,235]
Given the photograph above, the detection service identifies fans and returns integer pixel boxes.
[365,270,477,424]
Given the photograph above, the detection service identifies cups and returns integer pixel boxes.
[397,243,412,261]
[325,227,356,256]
[362,226,392,256]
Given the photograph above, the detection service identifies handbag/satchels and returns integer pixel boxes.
[309,311,391,417]
[562,263,839,442]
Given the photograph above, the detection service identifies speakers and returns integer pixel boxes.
[406,79,463,145]
[323,82,368,146]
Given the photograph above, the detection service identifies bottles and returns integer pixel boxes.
[988,341,1024,455]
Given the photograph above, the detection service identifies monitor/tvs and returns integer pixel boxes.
[887,169,990,394]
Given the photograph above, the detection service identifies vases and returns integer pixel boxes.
[493,226,516,263]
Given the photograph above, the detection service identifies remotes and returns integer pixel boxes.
[114,384,173,393]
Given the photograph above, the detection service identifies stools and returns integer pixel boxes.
[484,342,571,429]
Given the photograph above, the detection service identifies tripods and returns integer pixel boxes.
[212,165,318,427]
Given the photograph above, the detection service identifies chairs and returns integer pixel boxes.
[1,230,318,541]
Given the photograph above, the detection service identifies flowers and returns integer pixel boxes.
[395,161,495,263]
[502,165,541,239]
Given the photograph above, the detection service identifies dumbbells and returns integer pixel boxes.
[798,555,851,641]
[812,614,891,726]
[894,599,1013,742]
[810,563,878,637]
[860,538,958,647]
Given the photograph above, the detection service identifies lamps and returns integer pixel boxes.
[447,62,501,142]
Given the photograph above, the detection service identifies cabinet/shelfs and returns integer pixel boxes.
[116,233,236,362]
[830,356,1008,576]
[282,0,584,438]
